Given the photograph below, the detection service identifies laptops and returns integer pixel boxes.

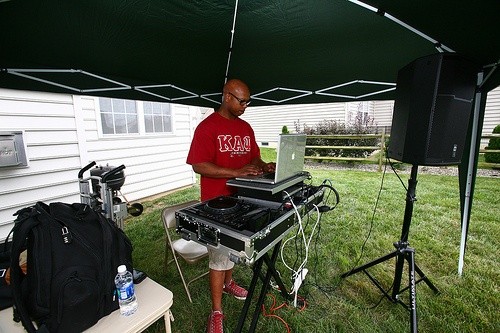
[235,134,306,184]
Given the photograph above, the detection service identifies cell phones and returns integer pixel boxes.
[133,269,147,284]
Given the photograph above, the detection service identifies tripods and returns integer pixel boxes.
[336,164,440,333]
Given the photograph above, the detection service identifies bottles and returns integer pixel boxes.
[114,265,138,316]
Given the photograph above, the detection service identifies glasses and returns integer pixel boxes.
[227,92,252,107]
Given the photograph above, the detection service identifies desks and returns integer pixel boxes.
[0,277,173,333]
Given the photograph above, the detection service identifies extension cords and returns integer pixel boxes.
[294,267,309,291]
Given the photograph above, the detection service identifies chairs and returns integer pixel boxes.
[161,200,209,304]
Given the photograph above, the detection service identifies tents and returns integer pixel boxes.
[0,0,500,278]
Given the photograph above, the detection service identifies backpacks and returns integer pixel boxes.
[2,202,134,333]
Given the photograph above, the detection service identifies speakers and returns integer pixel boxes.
[387,52,477,166]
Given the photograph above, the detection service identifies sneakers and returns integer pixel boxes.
[222,279,249,301]
[207,310,223,333]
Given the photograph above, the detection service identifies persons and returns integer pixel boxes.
[186,78,275,333]
